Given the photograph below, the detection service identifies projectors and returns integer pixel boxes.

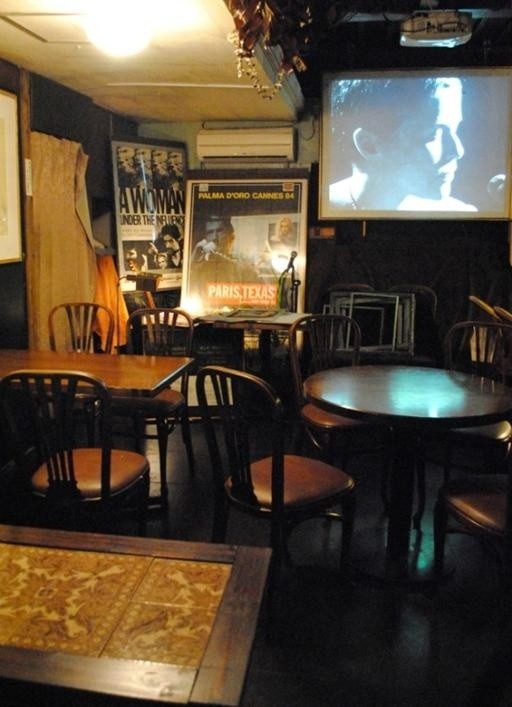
[398,10,473,49]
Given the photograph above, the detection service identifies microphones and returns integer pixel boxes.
[286,251,298,273]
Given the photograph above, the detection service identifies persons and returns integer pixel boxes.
[264,216,298,250]
[114,144,187,275]
[191,216,257,288]
[326,76,506,215]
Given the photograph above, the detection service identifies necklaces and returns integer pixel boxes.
[342,180,363,212]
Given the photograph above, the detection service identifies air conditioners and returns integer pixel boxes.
[196,125,299,163]
